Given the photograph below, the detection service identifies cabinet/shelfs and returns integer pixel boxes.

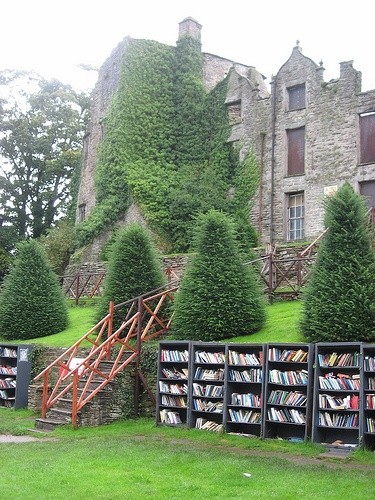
[358,344,375,450]
[189,341,227,432]
[0,343,34,410]
[155,340,191,429]
[311,340,364,442]
[223,343,267,438]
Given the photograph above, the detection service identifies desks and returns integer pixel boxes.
[260,343,315,439]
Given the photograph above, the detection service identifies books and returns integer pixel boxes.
[0,347,17,357]
[160,409,182,424]
[363,356,375,433]
[159,381,187,394]
[162,395,187,406]
[229,368,262,382]
[193,399,223,412]
[228,350,260,365]
[319,412,359,427]
[267,408,306,424]
[319,394,359,409]
[195,367,224,380]
[229,409,260,423]
[195,350,226,363]
[319,373,361,390]
[192,383,224,397]
[196,417,223,432]
[318,352,359,367]
[162,368,188,379]
[0,365,16,399]
[269,369,308,385]
[267,390,307,406]
[269,348,308,362]
[161,349,188,362]
[231,392,261,407]
